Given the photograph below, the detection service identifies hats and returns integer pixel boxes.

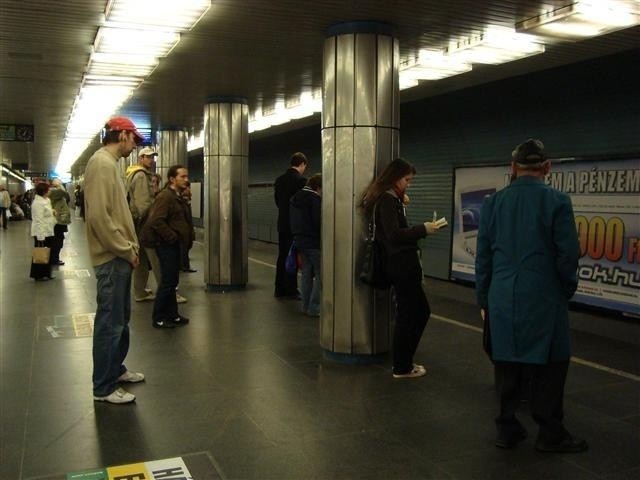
[511,142,547,168]
[138,148,158,157]
[107,117,144,143]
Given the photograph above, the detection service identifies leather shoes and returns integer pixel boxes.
[49,274,56,279]
[135,295,156,301]
[166,316,188,328]
[176,296,187,303]
[535,428,587,452]
[58,261,64,265]
[152,321,165,329]
[39,276,48,281]
[495,417,528,448]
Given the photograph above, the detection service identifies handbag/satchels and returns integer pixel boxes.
[359,242,380,289]
[285,240,297,274]
[32,241,50,264]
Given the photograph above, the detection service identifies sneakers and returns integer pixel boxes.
[117,370,144,382]
[392,364,424,372]
[93,387,136,404]
[393,368,426,378]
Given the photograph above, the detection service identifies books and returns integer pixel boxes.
[432,214,449,230]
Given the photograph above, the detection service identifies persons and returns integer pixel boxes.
[475,138,589,453]
[292,173,323,318]
[272,151,309,302]
[364,157,441,378]
[91,116,147,405]
[0,172,199,282]
[139,164,190,330]
[123,146,157,302]
